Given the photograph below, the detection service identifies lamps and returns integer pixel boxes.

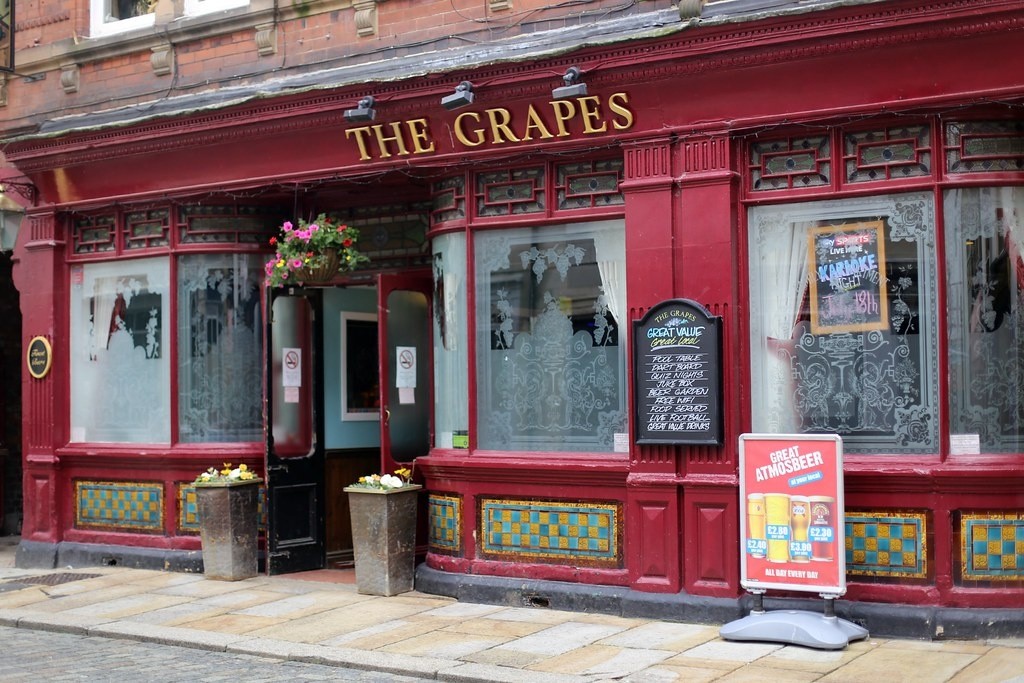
[343,96,375,123]
[550,65,587,100]
[441,79,477,111]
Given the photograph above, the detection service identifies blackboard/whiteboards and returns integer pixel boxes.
[634,298,722,445]
[808,220,888,335]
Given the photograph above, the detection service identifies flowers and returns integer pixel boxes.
[262,213,373,291]
[349,466,414,489]
[194,462,258,484]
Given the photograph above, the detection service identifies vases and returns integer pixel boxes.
[344,484,422,596]
[191,478,265,582]
[292,246,341,284]
